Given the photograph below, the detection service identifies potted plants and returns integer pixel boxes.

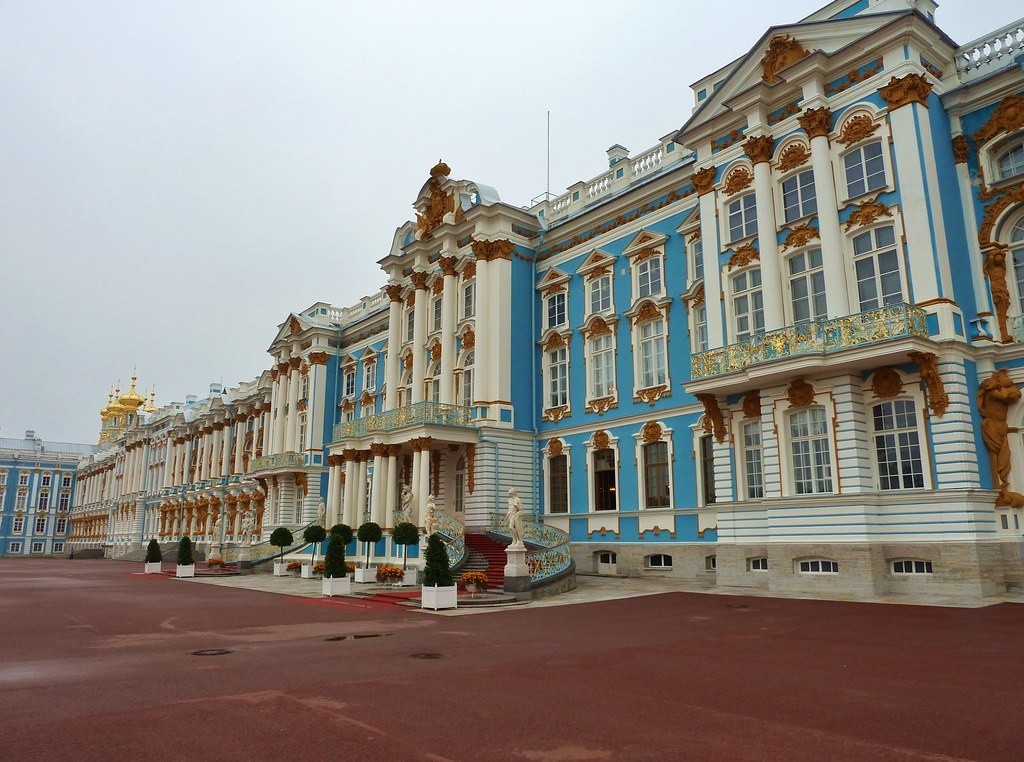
[377,565,404,583]
[269,524,357,597]
[461,571,488,592]
[176,536,196,578]
[207,557,225,569]
[355,522,382,584]
[144,539,163,574]
[391,522,421,587]
[421,534,458,610]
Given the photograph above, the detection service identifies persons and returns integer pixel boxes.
[157,491,265,533]
[424,494,436,536]
[401,486,414,524]
[241,511,253,545]
[212,514,224,544]
[987,250,1013,342]
[506,487,524,545]
[977,368,1021,484]
[315,496,326,528]
